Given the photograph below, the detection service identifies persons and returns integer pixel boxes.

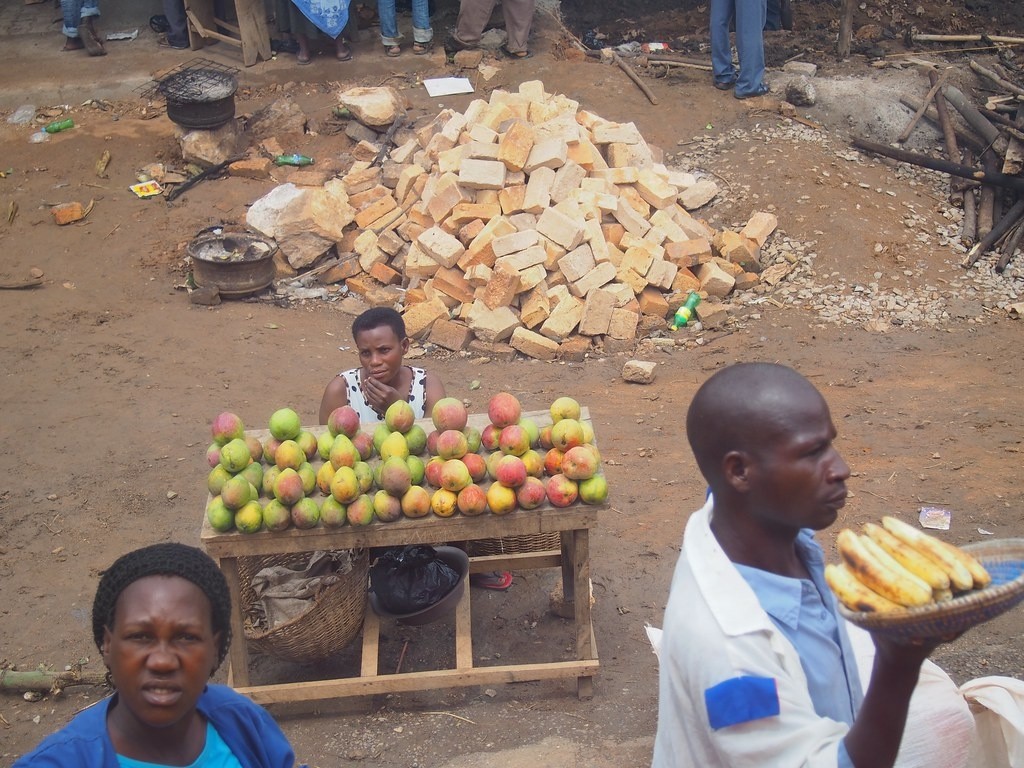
[378,0,435,57]
[276,0,355,64]
[11,542,308,768]
[709,0,770,99]
[320,306,513,590]
[62,0,108,57]
[444,0,534,63]
[649,362,977,768]
[155,0,229,49]
[764,0,791,31]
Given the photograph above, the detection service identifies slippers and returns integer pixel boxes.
[295,47,311,65]
[338,40,352,60]
[157,32,185,49]
[477,570,513,590]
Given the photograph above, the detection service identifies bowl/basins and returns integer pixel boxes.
[160,68,238,130]
[188,232,279,300]
[370,545,470,627]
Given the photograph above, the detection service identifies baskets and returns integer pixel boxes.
[836,538,1024,639]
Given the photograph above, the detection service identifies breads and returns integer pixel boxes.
[824,517,991,612]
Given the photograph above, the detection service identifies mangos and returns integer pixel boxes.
[207,391,607,534]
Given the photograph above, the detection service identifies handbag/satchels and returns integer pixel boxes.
[375,545,459,614]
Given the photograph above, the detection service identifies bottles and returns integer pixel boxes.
[276,155,315,166]
[670,291,701,331]
[41,118,74,133]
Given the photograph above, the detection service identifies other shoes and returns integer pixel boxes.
[499,41,532,60]
[715,69,740,90]
[734,81,770,99]
[384,45,401,57]
[449,37,465,52]
[149,14,171,34]
[413,38,435,55]
[271,37,299,53]
[77,25,107,56]
[63,37,84,50]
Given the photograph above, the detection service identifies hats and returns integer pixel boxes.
[92,541,234,680]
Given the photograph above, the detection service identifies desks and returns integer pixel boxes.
[200,405,612,711]
